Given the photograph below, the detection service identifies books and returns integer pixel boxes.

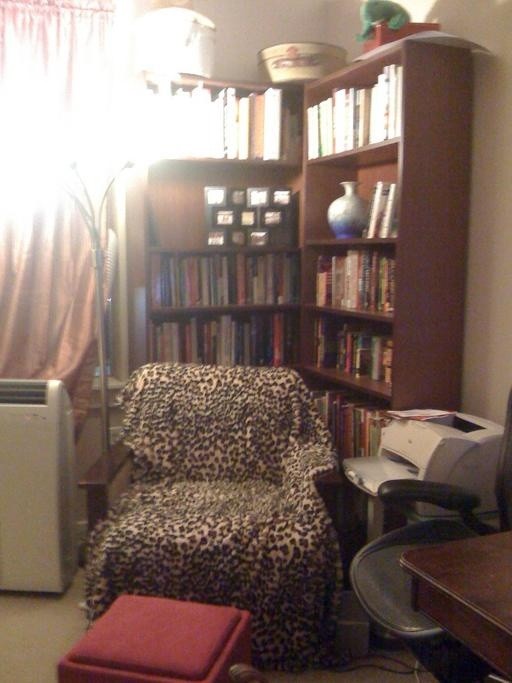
[307,66,403,160]
[315,247,396,313]
[149,252,296,308]
[333,477,387,546]
[311,387,391,467]
[312,314,393,384]
[159,82,292,161]
[147,311,296,369]
[361,181,402,239]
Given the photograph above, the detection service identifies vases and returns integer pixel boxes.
[327,181,367,237]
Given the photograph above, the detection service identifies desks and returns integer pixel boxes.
[399,533,512,680]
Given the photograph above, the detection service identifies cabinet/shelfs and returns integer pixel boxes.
[302,37,470,457]
[126,75,298,361]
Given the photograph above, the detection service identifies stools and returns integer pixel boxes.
[57,594,251,683]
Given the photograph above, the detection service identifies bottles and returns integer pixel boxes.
[324,180,373,241]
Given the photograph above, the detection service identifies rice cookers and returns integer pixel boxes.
[135,8,216,79]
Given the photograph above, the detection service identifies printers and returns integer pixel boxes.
[341,411,506,517]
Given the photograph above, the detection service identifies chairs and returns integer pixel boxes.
[78,360,338,670]
[349,390,512,683]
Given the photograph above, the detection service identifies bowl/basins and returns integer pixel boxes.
[257,41,349,85]
